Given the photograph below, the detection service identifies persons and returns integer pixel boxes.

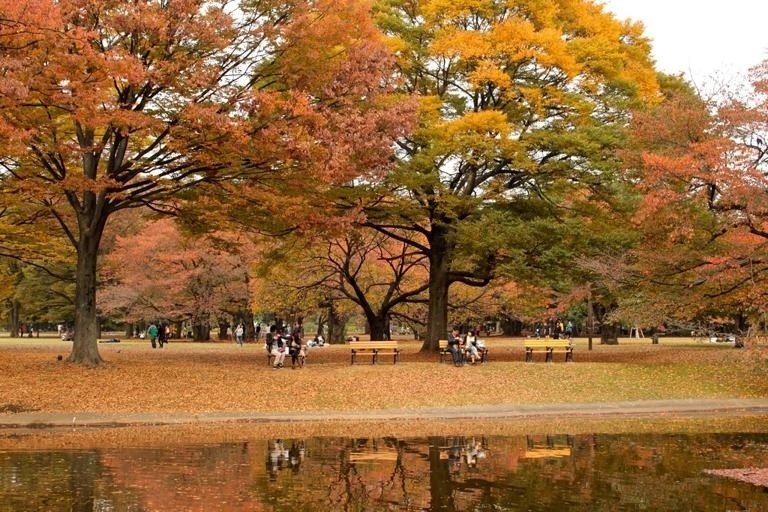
[286,440,304,475]
[267,438,288,482]
[461,330,482,364]
[16,320,74,341]
[443,329,464,367]
[457,319,574,339]
[265,325,286,368]
[288,333,304,369]
[445,432,463,470]
[465,435,485,476]
[132,321,361,350]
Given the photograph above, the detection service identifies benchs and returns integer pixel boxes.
[265,346,307,369]
[438,339,489,363]
[348,340,400,365]
[521,337,576,362]
